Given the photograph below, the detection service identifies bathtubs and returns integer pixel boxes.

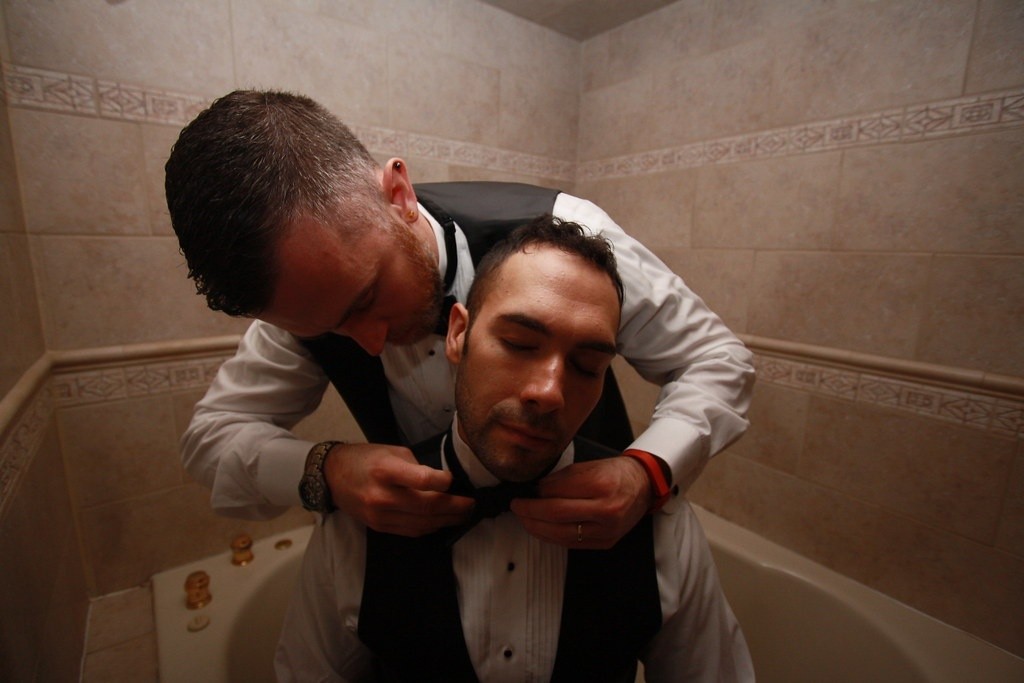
[151,501,1022,682]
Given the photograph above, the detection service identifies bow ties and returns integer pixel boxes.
[444,420,561,519]
[417,194,458,336]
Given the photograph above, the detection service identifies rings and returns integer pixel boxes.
[577,525,583,543]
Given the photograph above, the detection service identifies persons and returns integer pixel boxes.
[273,215,756,683]
[164,90,760,551]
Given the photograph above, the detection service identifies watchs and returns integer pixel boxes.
[298,441,343,515]
